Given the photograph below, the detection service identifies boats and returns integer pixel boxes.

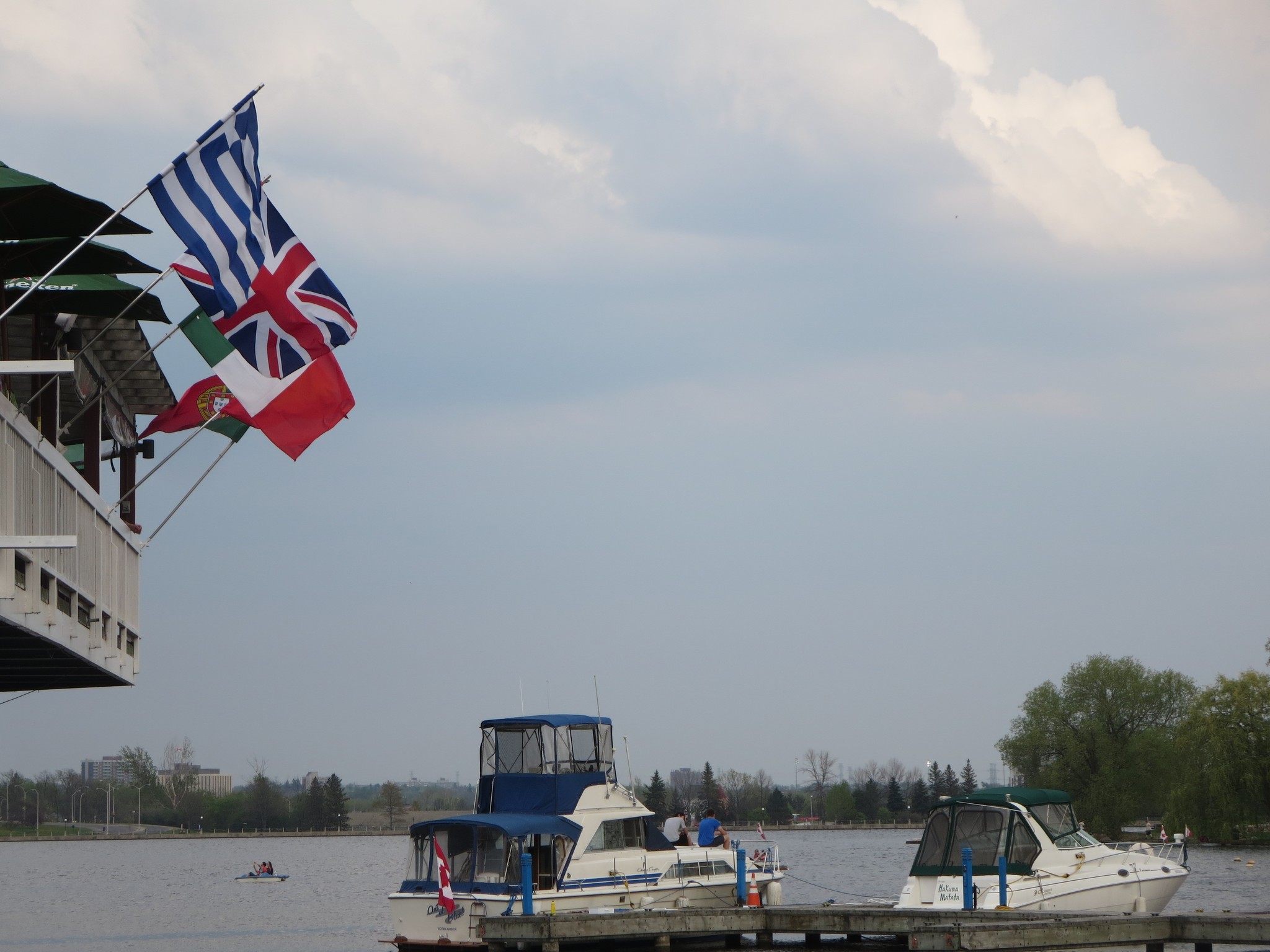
[234,872,289,882]
[898,785,1191,915]
[376,670,786,952]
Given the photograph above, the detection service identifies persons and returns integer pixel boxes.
[1078,820,1085,830]
[754,849,767,863]
[664,811,692,846]
[697,809,730,850]
[1145,820,1153,839]
[246,861,273,876]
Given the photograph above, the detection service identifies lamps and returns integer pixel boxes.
[138,439,156,459]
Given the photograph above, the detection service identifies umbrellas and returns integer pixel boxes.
[0,159,172,326]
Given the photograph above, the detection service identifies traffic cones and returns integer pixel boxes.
[746,872,765,909]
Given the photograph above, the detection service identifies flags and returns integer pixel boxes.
[435,839,456,915]
[146,89,267,319]
[178,299,356,461]
[1159,827,1168,842]
[168,184,357,379]
[137,375,350,445]
[1185,827,1194,838]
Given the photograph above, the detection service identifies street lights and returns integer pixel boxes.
[64,819,67,830]
[810,795,813,823]
[80,792,86,835]
[99,779,122,823]
[14,785,35,837]
[74,786,89,820]
[96,788,119,835]
[106,784,121,824]
[71,790,81,821]
[795,758,799,785]
[32,789,38,837]
[128,784,151,830]
